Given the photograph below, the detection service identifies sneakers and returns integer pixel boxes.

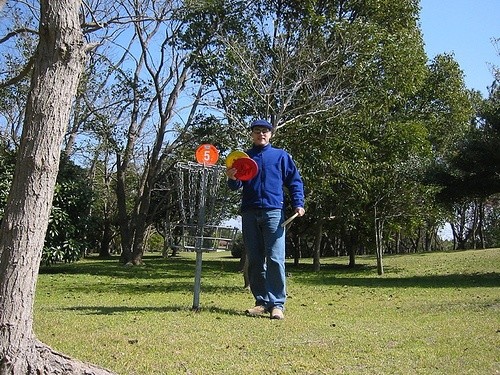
[270,307,285,320]
[245,305,270,315]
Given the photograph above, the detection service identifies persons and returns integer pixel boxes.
[225,119,306,319]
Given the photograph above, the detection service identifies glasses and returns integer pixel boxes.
[251,127,270,134]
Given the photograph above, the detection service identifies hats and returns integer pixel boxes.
[251,120,274,131]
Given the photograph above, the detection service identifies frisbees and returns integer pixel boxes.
[232,157,258,181]
[226,150,249,169]
[196,144,219,165]
[281,212,299,228]
